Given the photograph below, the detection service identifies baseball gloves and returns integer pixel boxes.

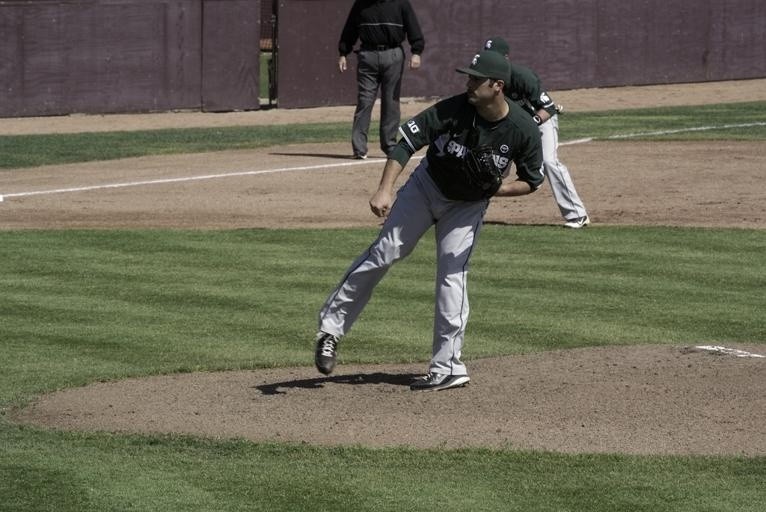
[457,145,501,202]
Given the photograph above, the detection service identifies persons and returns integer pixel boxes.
[313,50,547,391]
[482,36,590,230]
[337,0,425,160]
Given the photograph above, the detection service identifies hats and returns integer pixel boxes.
[456,50,511,84]
[484,37,510,56]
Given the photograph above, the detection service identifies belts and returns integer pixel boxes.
[373,44,396,51]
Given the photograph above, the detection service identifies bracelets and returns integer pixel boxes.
[532,114,543,125]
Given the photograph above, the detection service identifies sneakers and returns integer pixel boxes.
[354,154,367,159]
[410,371,470,393]
[564,216,590,228]
[315,331,337,373]
